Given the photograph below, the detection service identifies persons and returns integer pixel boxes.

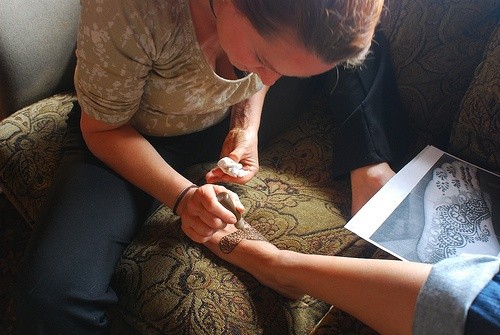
[36,0,398,335]
[202,218,500,335]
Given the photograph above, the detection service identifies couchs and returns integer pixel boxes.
[0,0,500,335]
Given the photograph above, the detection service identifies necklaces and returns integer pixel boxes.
[208,1,218,20]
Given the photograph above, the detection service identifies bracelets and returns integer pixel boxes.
[172,185,199,216]
